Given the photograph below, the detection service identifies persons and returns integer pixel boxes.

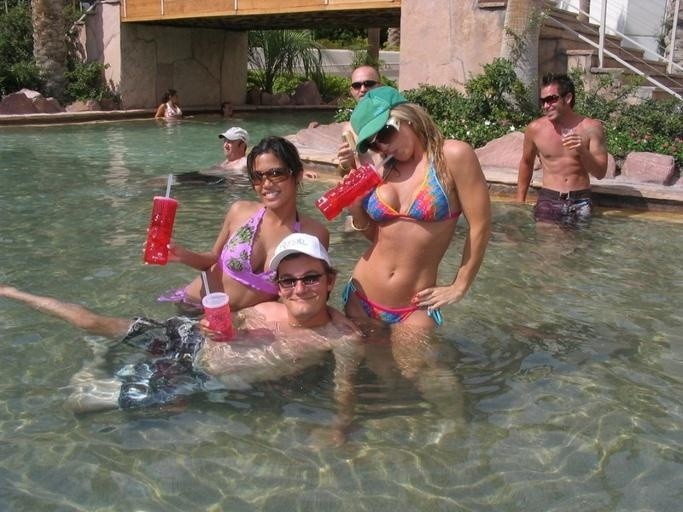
[154,88,194,122]
[515,70,609,252]
[341,85,494,446]
[220,101,232,116]
[144,126,318,193]
[0,231,368,458]
[333,63,383,175]
[140,134,331,317]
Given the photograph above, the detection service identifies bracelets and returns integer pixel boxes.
[351,217,371,233]
[337,164,353,171]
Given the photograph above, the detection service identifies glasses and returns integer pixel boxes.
[367,116,401,154]
[277,272,324,288]
[351,80,378,89]
[248,166,293,186]
[539,95,560,106]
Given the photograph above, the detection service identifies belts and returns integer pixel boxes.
[558,191,570,200]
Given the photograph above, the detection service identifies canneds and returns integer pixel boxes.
[342,131,355,151]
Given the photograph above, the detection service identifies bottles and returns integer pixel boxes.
[202,292,235,341]
[143,195,178,266]
[315,162,383,221]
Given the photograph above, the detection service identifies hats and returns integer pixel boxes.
[218,126,249,145]
[350,85,408,155]
[269,233,331,272]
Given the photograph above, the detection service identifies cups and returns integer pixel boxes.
[560,127,574,150]
